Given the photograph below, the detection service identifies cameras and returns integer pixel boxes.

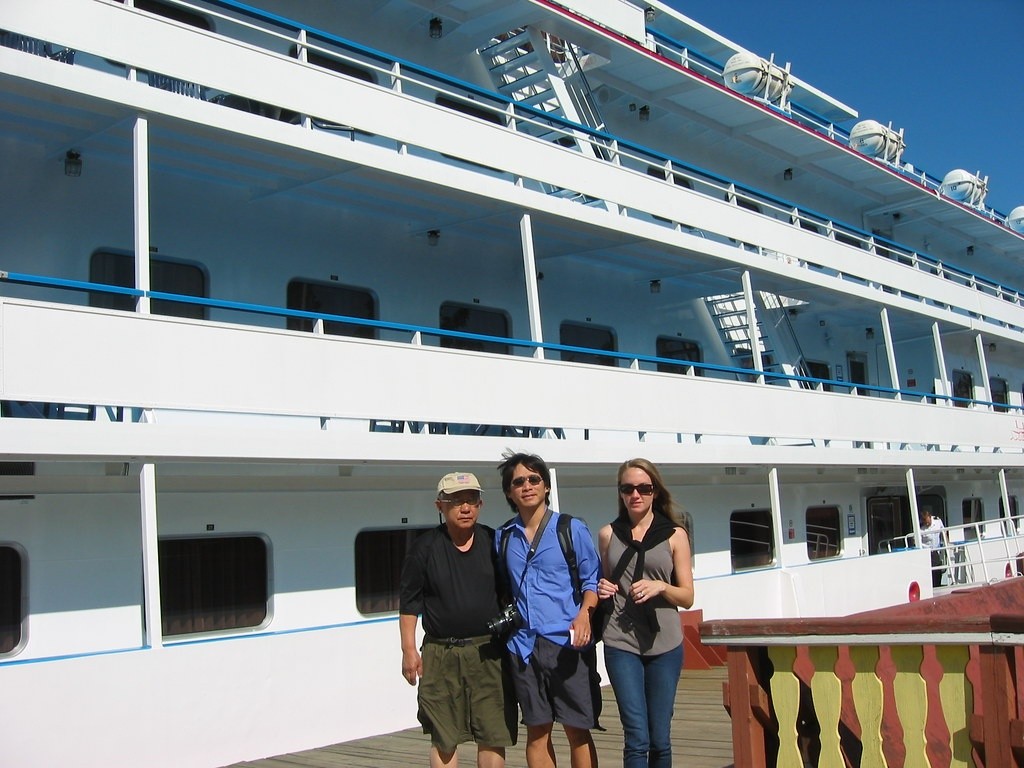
[485,604,524,644]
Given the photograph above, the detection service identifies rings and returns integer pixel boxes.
[584,635,588,637]
[639,591,643,597]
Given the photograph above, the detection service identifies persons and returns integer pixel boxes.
[400,471,518,768]
[598,458,694,768]
[495,448,602,768]
[912,511,948,587]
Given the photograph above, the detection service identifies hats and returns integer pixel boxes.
[436,472,484,500]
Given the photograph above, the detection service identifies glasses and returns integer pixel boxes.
[440,493,482,506]
[618,483,654,495]
[509,475,543,487]
